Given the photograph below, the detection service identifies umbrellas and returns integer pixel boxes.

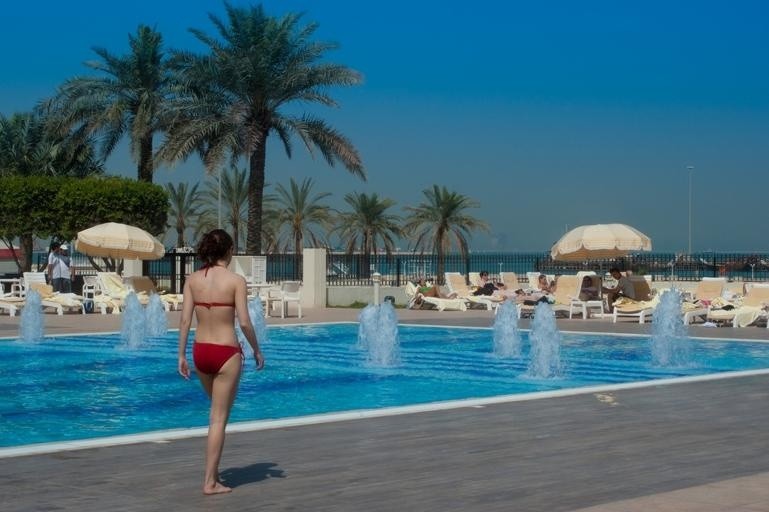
[550,222,653,276]
[74,220,166,277]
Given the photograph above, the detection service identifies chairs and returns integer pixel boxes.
[467,286,519,311]
[625,276,651,300]
[612,297,656,324]
[553,276,602,311]
[680,280,724,325]
[421,290,472,312]
[500,271,519,285]
[526,271,541,286]
[266,279,302,318]
[445,272,460,292]
[706,286,768,326]
[0,268,183,317]
[468,272,480,283]
[518,275,576,319]
[576,271,597,289]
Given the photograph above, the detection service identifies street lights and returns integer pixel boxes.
[685,164,694,265]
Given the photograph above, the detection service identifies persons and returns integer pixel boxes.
[48,244,76,294]
[414,278,458,304]
[479,271,526,305]
[601,268,637,314]
[538,275,558,294]
[569,275,597,308]
[496,282,548,305]
[516,289,547,299]
[176,228,264,495]
[47,242,62,293]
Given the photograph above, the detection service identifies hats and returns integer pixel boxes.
[60,245,68,250]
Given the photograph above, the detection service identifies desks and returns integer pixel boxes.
[247,284,275,310]
[569,300,605,320]
